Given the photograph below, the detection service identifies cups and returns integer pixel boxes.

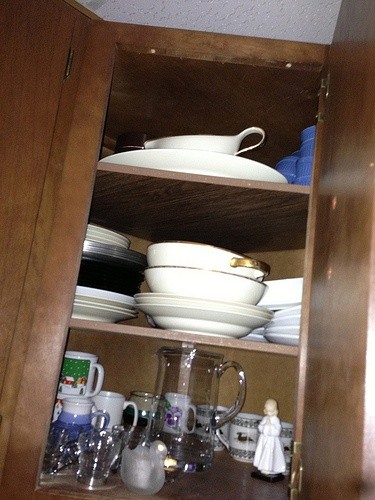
[216,412,264,463]
[277,126,317,185]
[196,405,230,451]
[41,351,168,496]
[161,392,197,434]
[279,420,294,472]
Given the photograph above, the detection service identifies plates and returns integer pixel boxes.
[70,223,148,323]
[134,293,301,346]
[99,149,289,184]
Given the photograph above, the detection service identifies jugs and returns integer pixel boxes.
[144,126,266,156]
[143,348,246,472]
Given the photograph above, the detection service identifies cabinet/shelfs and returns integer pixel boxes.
[0,0,375,500]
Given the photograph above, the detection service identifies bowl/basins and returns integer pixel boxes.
[144,241,271,306]
